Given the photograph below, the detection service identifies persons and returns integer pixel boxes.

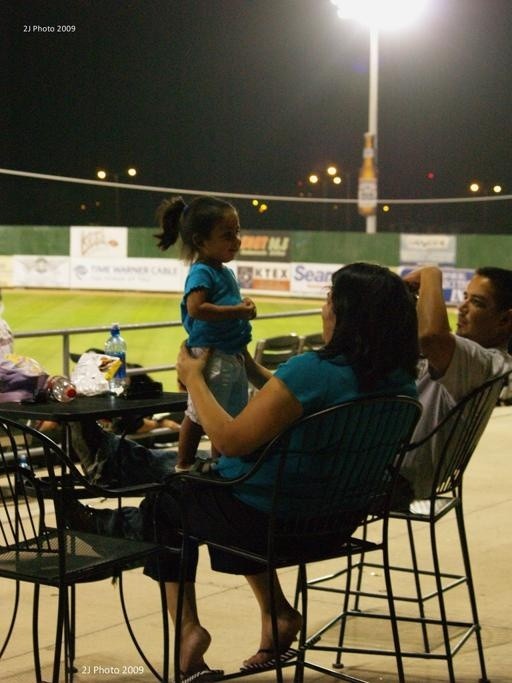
[153,194,257,474]
[136,261,417,679]
[38,346,158,433]
[372,264,510,509]
[1,295,16,360]
[163,377,188,429]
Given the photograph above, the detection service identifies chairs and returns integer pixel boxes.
[0,331,512,682]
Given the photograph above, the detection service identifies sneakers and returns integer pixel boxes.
[175,456,215,474]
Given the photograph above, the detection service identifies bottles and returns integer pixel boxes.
[356,130,385,216]
[48,370,78,403]
[105,322,127,396]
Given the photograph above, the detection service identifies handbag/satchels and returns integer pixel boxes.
[0,354,48,403]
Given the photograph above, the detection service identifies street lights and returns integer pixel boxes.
[327,164,352,232]
[97,167,139,226]
[311,174,328,232]
[328,0,428,234]
[467,184,502,234]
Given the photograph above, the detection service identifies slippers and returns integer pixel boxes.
[241,635,322,668]
[179,663,225,682]
